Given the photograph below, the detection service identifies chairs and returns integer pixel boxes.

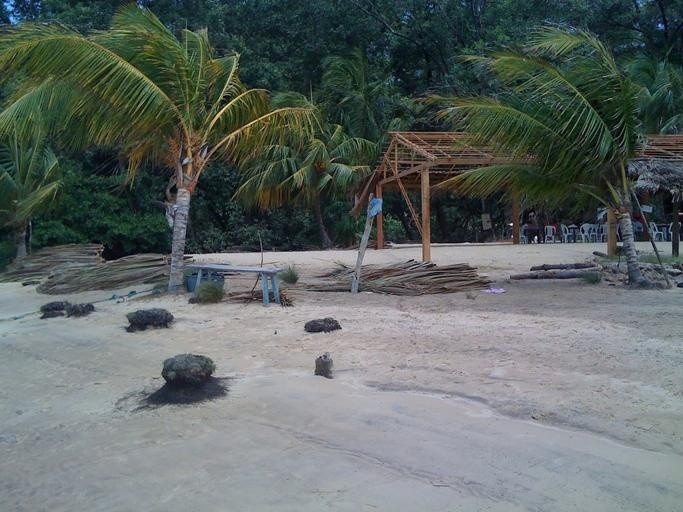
[521,222,682,244]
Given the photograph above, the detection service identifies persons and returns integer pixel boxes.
[521,220,533,244]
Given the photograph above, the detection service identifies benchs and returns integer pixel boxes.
[185,263,284,305]
